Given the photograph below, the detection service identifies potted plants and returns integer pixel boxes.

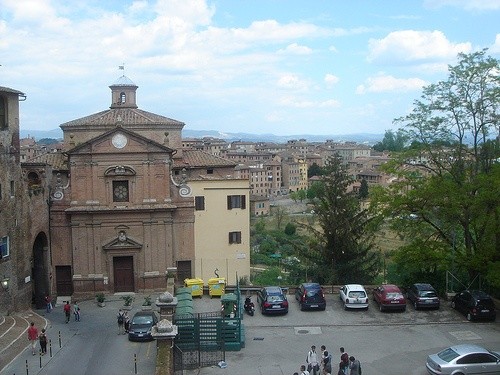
[121,295,135,311]
[445,289,456,299]
[96,293,105,307]
[142,295,154,311]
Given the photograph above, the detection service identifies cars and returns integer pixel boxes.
[452,289,497,322]
[373,284,406,312]
[128,311,158,341]
[425,343,500,375]
[256,285,289,315]
[339,284,369,311]
[294,282,327,311]
[405,283,440,309]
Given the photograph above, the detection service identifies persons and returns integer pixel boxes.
[349,356,361,375]
[293,351,331,375]
[338,362,346,375]
[319,345,326,375]
[44,293,51,313]
[64,301,70,321]
[117,310,124,329]
[38,329,48,354]
[74,302,80,321]
[306,345,319,375]
[340,347,348,369]
[28,322,39,355]
[125,311,129,332]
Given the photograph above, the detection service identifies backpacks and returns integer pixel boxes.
[118,312,124,325]
[39,333,47,343]
[306,351,316,363]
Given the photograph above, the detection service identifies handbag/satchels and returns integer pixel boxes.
[28,336,32,340]
[49,302,54,311]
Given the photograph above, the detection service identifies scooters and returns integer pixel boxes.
[243,296,255,316]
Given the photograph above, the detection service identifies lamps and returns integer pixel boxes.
[164,130,169,137]
[69,133,74,139]
